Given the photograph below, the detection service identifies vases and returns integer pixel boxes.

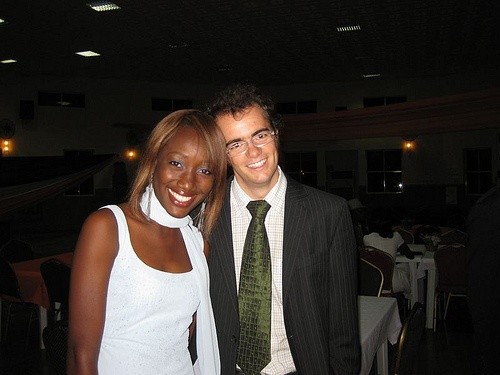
[422,241,439,252]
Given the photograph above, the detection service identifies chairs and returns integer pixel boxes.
[357,228,469,374]
[0,259,73,375]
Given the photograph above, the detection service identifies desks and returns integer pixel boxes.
[395,244,443,329]
[1,252,74,370]
[357,296,402,375]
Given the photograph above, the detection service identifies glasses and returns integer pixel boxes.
[226,128,278,157]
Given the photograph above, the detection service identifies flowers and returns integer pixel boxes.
[421,232,441,247]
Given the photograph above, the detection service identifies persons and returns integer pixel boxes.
[188,83,361,375]
[362,208,425,323]
[67,110,227,375]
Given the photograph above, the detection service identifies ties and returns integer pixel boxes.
[237,200,272,375]
